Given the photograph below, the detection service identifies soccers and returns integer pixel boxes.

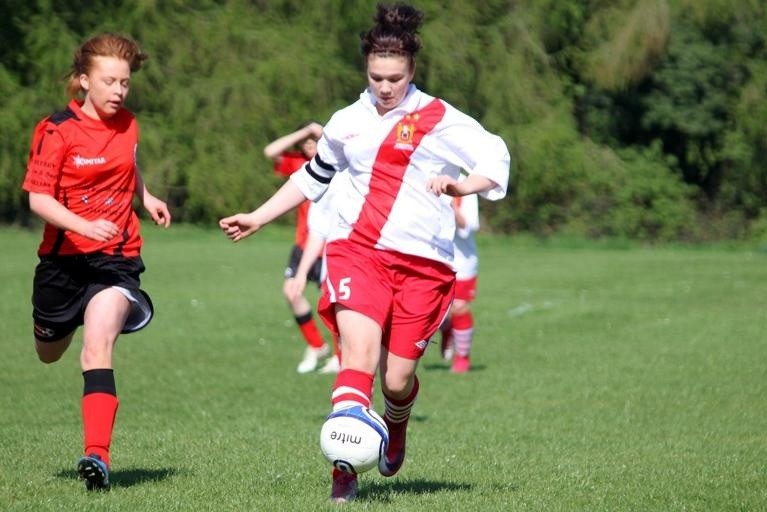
[321,407,387,471]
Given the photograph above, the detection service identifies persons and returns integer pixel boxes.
[263,119,346,374]
[219,3,511,502]
[439,172,482,374]
[22,36,171,490]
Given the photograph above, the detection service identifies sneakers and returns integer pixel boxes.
[317,354,340,373]
[377,414,408,477]
[451,350,469,372]
[441,321,453,360]
[297,342,330,373]
[77,452,110,492]
[331,468,359,503]
